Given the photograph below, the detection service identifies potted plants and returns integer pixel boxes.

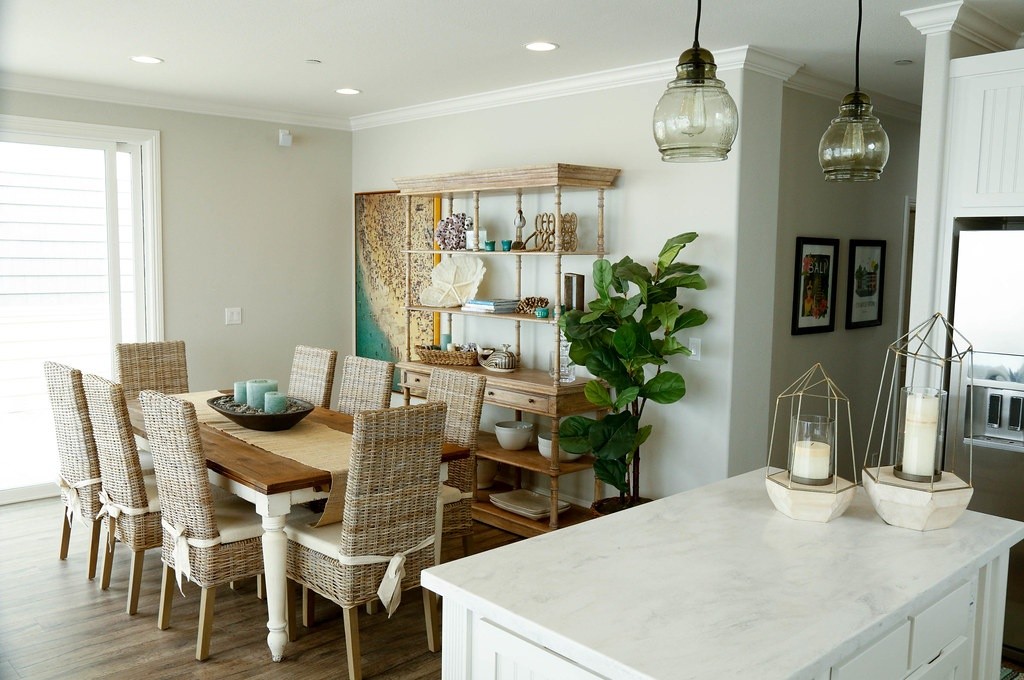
[558,232,710,519]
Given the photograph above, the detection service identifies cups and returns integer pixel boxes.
[549,351,555,377]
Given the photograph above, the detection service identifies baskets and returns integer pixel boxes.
[414,344,495,366]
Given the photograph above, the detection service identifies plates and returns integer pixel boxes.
[490,489,570,520]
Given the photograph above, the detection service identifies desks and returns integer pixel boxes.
[126,389,471,662]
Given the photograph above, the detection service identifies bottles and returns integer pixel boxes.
[560,339,575,382]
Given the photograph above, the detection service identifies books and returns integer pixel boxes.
[462,299,518,314]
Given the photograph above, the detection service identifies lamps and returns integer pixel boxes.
[817,0,890,183]
[652,0,738,164]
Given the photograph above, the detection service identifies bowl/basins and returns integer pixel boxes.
[538,432,582,461]
[207,395,314,431]
[495,421,534,450]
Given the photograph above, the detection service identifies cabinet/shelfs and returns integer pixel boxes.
[420,465,1024,680]
[393,161,622,539]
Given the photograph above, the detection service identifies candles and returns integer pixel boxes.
[234,381,247,403]
[264,391,286,415]
[787,414,835,487]
[892,385,949,483]
[247,379,278,409]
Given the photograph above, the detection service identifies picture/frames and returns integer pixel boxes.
[790,235,839,336]
[844,239,887,331]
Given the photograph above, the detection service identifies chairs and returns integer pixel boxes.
[287,342,338,408]
[41,360,156,581]
[114,340,190,452]
[283,400,448,680]
[138,387,316,663]
[337,355,396,417]
[81,371,243,617]
[426,366,487,557]
